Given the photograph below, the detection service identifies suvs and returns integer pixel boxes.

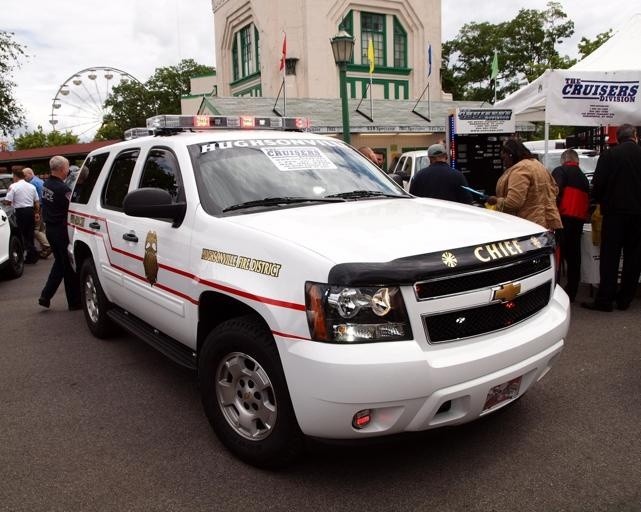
[64,112,578,461]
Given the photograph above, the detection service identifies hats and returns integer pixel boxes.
[427,144,448,158]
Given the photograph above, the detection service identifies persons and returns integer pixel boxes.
[3,165,51,264]
[38,156,86,310]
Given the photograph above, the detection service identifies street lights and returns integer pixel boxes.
[327,21,357,146]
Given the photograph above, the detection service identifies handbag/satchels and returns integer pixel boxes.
[590,208,604,248]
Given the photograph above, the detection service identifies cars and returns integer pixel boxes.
[387,136,610,196]
[0,167,78,285]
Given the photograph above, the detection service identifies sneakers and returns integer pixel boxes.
[68,303,83,312]
[39,297,51,309]
[618,295,634,310]
[581,301,614,312]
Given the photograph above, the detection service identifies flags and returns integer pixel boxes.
[427,47,433,77]
[366,36,375,75]
[491,53,499,81]
[279,33,289,71]
